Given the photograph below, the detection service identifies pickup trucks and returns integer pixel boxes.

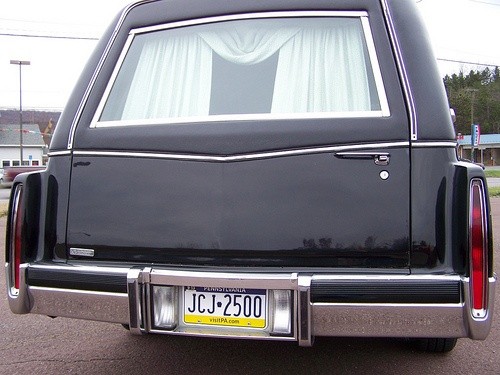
[6,166,46,185]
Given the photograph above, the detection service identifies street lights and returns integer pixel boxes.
[10,60,31,166]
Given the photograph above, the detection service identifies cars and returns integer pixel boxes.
[0,168,5,183]
[4,1,495,356]
